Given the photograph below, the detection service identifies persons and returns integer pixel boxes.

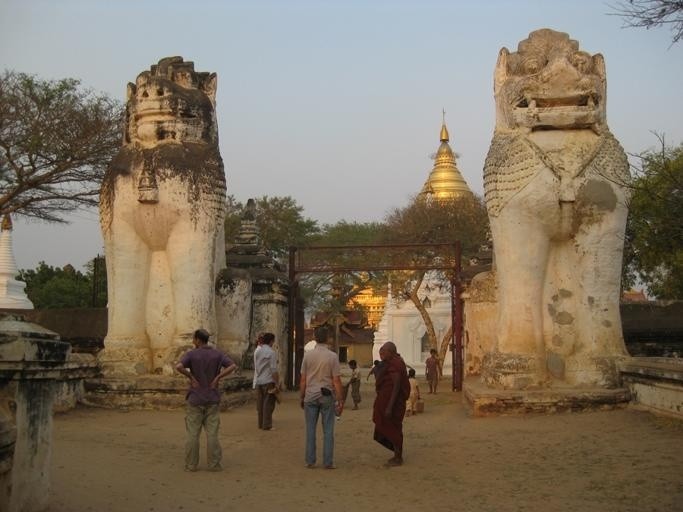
[372,341,411,466]
[249,332,443,431]
[175,328,236,472]
[300,326,344,469]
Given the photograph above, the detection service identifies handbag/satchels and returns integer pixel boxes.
[321,387,332,396]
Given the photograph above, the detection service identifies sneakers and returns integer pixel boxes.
[384,457,404,466]
